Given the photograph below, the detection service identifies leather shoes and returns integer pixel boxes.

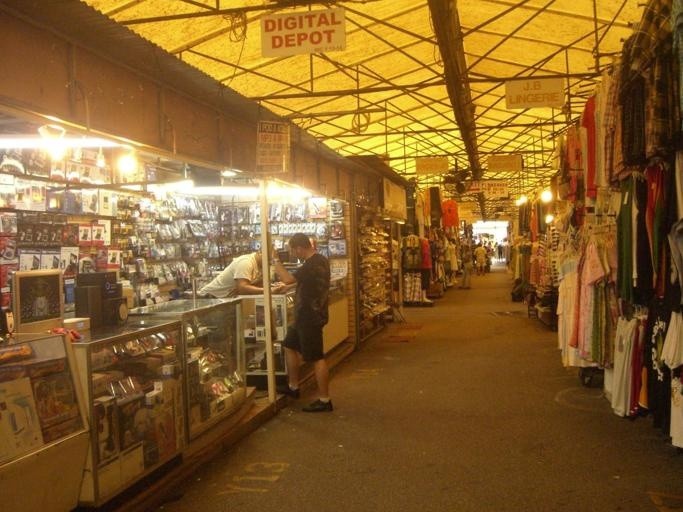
[302,399,333,412]
[276,385,300,398]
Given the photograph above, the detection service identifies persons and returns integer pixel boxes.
[272,233,333,412]
[434,231,506,290]
[198,246,295,298]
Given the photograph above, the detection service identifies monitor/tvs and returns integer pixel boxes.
[13,269,64,343]
[190,166,222,187]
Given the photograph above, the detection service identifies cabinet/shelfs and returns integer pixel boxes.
[237,275,346,378]
[356,207,393,342]
[0,170,352,309]
[2,330,90,509]
[136,296,248,442]
[78,316,184,508]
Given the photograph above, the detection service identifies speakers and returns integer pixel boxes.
[104,297,129,329]
[74,284,101,325]
[0,307,15,335]
